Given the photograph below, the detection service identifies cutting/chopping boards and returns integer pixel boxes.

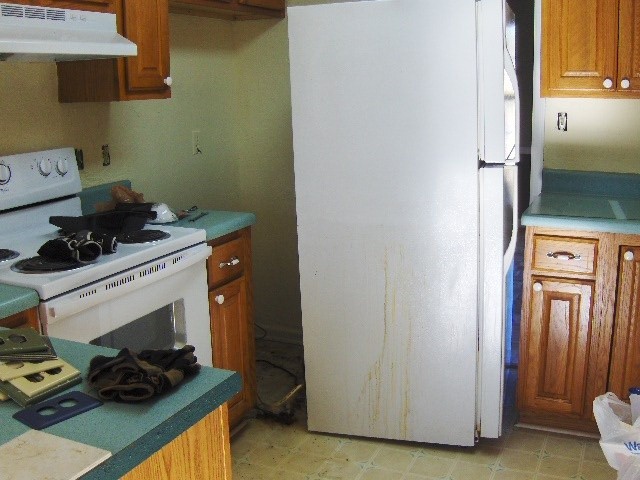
[0,428,111,476]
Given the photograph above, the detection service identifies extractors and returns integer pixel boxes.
[0,0,138,64]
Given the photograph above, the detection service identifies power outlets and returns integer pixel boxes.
[194,132,203,159]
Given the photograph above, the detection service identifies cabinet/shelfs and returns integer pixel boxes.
[607,230,640,403]
[522,229,601,429]
[542,2,639,101]
[58,1,173,102]
[211,220,259,442]
[8,312,38,333]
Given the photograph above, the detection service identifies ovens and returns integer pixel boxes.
[39,242,215,368]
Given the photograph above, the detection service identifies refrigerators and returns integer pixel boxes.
[286,0,522,448]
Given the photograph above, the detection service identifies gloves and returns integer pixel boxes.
[89,345,200,400]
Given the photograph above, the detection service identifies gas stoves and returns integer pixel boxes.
[0,222,172,276]
